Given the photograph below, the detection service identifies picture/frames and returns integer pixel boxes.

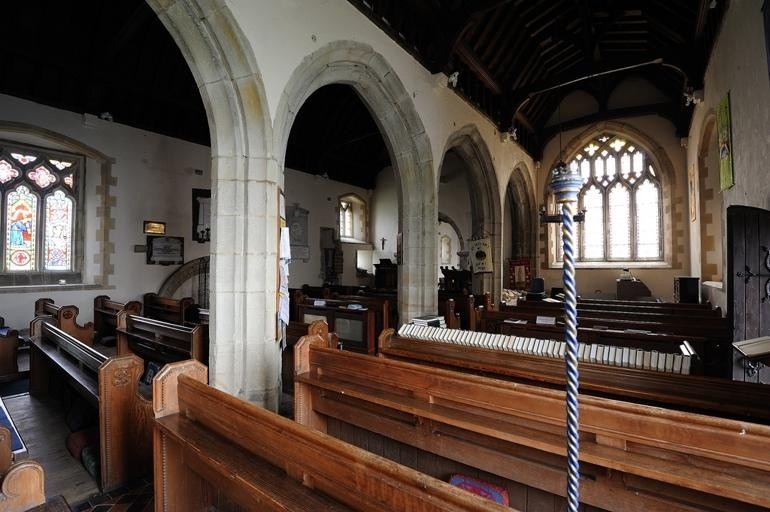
[146,235,186,265]
[192,187,212,242]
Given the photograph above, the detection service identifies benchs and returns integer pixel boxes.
[0,276,769,511]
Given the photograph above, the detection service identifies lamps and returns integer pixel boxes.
[551,85,572,175]
